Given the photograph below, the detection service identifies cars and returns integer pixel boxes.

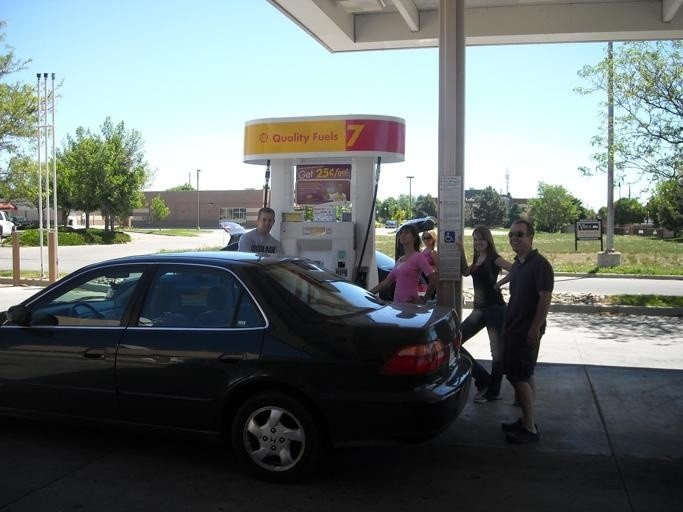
[10,215,33,230]
[384,220,396,229]
[420,216,437,225]
[215,218,438,306]
[0,248,474,488]
[0,210,17,238]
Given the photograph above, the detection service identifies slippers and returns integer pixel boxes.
[489,396,502,400]
[502,417,538,431]
[473,385,487,403]
[505,424,540,443]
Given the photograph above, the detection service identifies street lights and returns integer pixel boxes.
[196,168,202,230]
[404,176,416,218]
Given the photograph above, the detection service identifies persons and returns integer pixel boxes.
[455,226,512,405]
[420,229,439,294]
[238,207,285,256]
[328,183,346,203]
[501,219,554,443]
[368,225,437,305]
[444,232,454,243]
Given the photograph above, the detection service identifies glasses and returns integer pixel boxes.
[508,231,527,237]
